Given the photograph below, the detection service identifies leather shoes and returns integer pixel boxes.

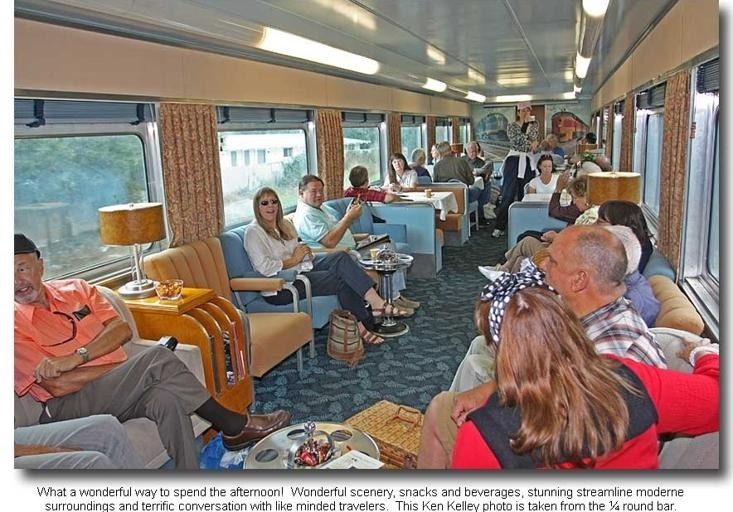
[221,409,291,452]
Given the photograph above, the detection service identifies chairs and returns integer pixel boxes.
[13,141,721,469]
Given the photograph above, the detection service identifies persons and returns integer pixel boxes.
[448,224,661,393]
[344,168,405,202]
[292,176,420,318]
[13,412,149,473]
[446,286,721,471]
[13,232,291,472]
[383,103,662,330]
[418,224,667,470]
[242,186,407,346]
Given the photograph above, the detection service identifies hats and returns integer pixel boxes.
[14,233,41,259]
[517,101,533,111]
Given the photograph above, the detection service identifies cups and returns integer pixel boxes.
[425,189,431,199]
[370,249,380,262]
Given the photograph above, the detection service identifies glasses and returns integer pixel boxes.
[40,311,77,348]
[258,200,279,205]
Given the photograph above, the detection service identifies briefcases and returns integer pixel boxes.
[341,398,425,470]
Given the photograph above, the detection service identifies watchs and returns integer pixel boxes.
[75,347,89,362]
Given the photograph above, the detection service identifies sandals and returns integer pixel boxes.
[371,302,409,318]
[360,328,385,345]
[484,263,509,273]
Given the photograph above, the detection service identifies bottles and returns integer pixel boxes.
[297,237,313,271]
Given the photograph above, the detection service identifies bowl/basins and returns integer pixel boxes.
[154,280,182,301]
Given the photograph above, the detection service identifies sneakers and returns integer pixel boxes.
[491,228,505,238]
[392,296,421,318]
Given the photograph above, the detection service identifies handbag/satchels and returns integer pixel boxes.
[326,309,365,368]
